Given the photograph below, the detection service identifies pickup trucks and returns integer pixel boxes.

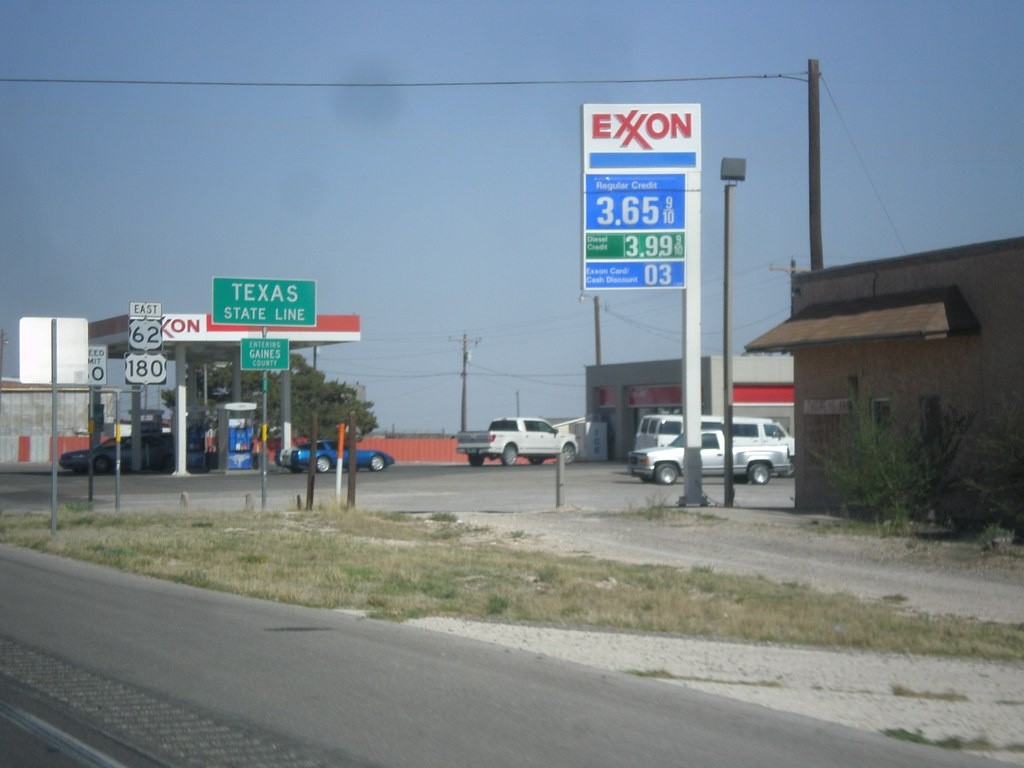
[455,417,581,466]
[629,428,792,486]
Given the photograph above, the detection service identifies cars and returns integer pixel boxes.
[59,430,175,475]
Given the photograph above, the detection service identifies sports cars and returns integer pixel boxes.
[275,439,396,472]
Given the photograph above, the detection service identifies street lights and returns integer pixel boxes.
[721,154,748,509]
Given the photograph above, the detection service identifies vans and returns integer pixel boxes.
[632,415,796,479]
